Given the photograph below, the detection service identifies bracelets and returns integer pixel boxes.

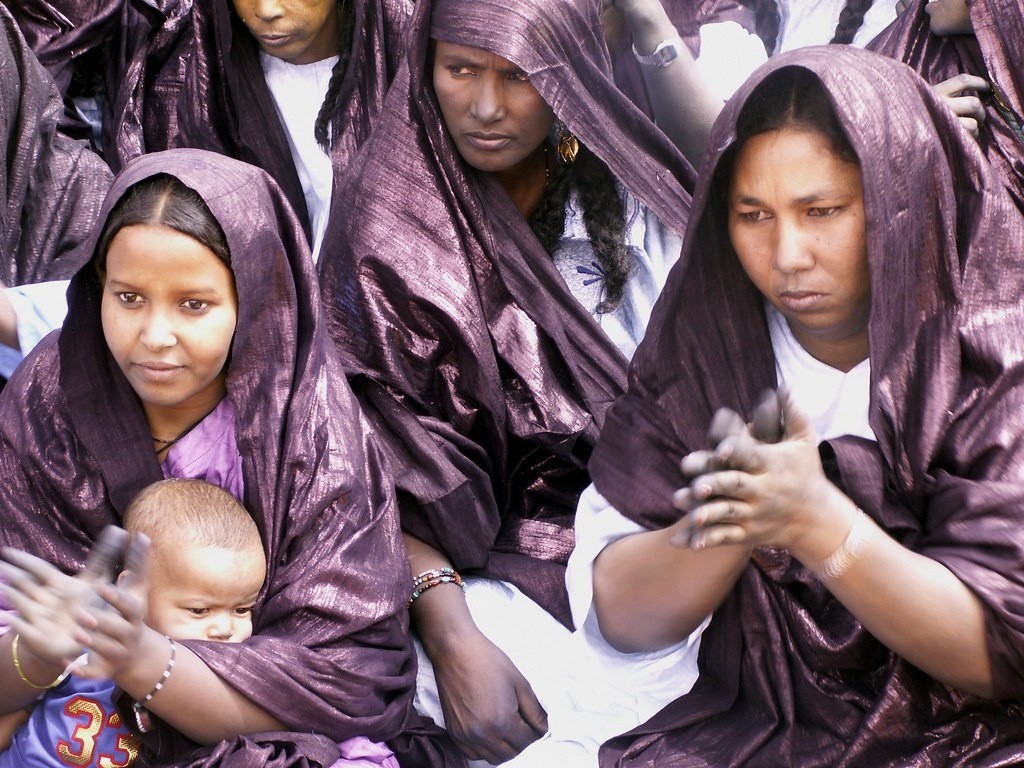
[9,631,75,690]
[406,573,464,600]
[397,576,466,617]
[127,626,177,708]
[407,566,461,585]
[806,506,877,589]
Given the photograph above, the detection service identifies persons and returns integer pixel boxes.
[563,37,1024,768]
[0,5,129,395]
[764,0,913,70]
[2,477,268,767]
[319,0,760,768]
[0,141,422,767]
[6,0,170,183]
[866,0,1024,214]
[145,0,419,360]
[593,0,770,183]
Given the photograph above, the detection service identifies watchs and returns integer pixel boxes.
[627,26,685,70]
